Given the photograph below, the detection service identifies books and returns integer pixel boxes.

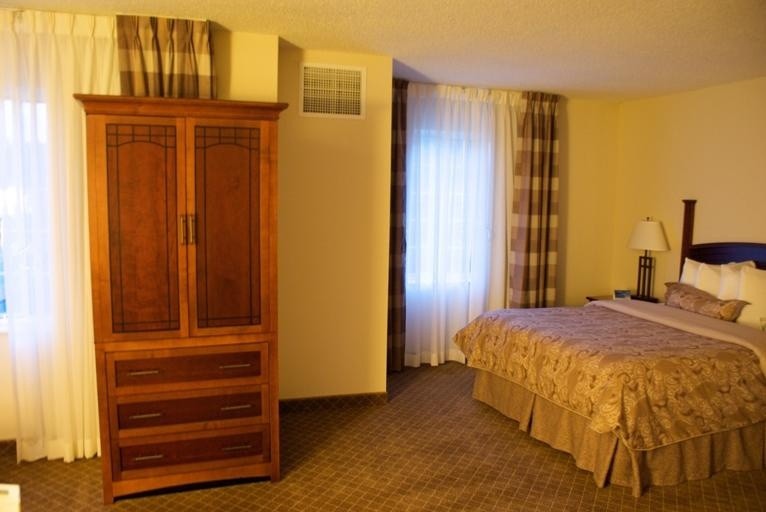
[612,287,633,301]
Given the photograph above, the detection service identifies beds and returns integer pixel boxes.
[476,199,766,488]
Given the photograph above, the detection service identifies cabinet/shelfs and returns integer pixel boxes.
[72,93,289,504]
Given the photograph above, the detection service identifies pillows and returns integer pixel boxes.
[663,257,766,333]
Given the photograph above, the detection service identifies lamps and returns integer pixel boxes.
[626,217,670,304]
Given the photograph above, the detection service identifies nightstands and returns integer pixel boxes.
[585,294,613,304]
